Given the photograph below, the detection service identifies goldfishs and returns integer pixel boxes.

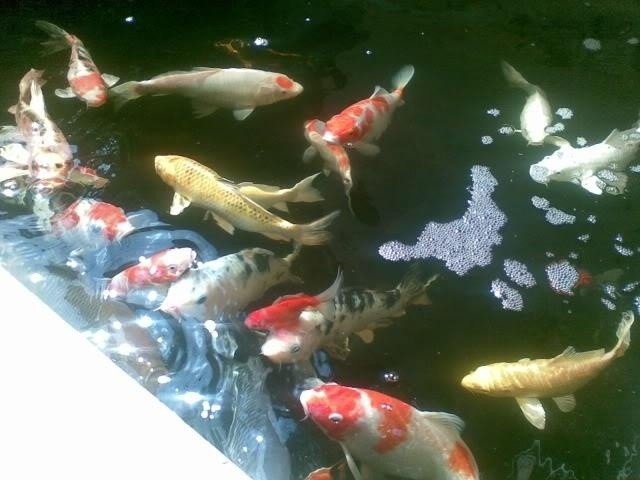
[74,246,480,479]
